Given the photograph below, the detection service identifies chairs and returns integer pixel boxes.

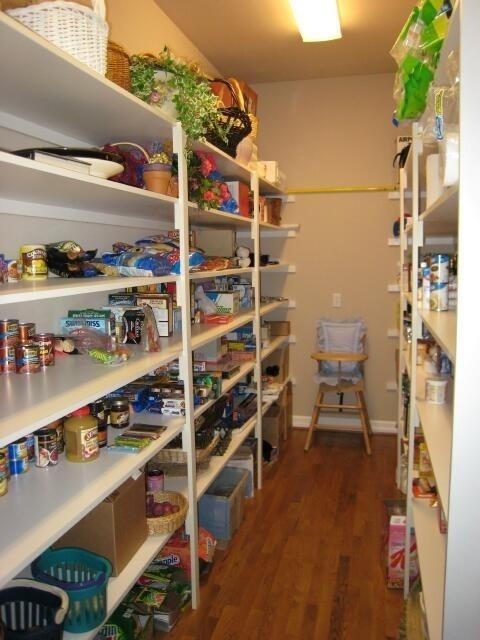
[303,323,373,455]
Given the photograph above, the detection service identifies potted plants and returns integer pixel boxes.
[128,46,227,164]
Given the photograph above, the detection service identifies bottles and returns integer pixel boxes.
[64,406,100,463]
[92,399,105,424]
[104,401,111,426]
[46,418,65,454]
[98,419,108,448]
[110,397,130,429]
[33,428,58,468]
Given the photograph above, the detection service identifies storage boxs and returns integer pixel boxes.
[50,471,148,576]
[381,499,418,588]
[262,408,284,448]
[198,466,250,542]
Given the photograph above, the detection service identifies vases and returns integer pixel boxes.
[141,171,172,195]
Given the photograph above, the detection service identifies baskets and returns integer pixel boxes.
[7,0,109,81]
[107,37,133,90]
[146,490,189,536]
[154,437,220,468]
[202,75,251,155]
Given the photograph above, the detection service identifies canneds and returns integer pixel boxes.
[0,346,16,374]
[8,437,27,459]
[19,244,48,280]
[33,333,56,367]
[417,339,427,364]
[0,446,10,497]
[14,342,42,374]
[146,470,164,491]
[24,434,35,461]
[19,323,37,343]
[0,319,19,348]
[430,285,450,312]
[429,253,451,284]
[9,456,29,475]
[426,378,447,404]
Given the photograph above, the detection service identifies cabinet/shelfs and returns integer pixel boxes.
[2,10,291,640]
[392,4,480,640]
